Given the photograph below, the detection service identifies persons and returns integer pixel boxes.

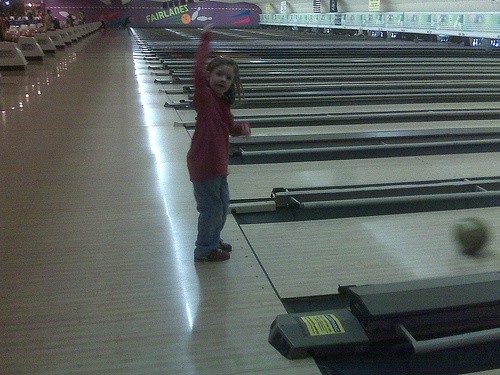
[0,0,87,41]
[98,12,107,29]
[186,21,252,264]
[122,15,133,36]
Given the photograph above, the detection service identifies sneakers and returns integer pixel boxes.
[207,243,232,261]
